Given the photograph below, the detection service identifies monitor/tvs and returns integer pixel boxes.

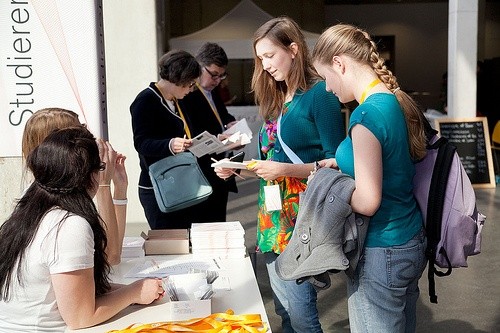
[220,58,260,107]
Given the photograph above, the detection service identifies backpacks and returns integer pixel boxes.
[412,102,487,304]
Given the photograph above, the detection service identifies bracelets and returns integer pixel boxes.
[112,198,127,205]
[98,184,110,187]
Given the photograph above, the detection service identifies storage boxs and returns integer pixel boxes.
[140,229,189,254]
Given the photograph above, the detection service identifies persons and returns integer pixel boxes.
[214,17,346,333]
[130,51,229,231]
[312,24,428,333]
[0,126,165,333]
[22,107,129,265]
[176,41,242,223]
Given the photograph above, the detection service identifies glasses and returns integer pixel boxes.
[204,67,227,81]
[188,81,196,88]
[82,123,88,128]
[95,161,106,171]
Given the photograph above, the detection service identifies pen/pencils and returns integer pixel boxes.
[180,134,189,154]
[210,156,246,182]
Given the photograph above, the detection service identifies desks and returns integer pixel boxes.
[65,250,272,333]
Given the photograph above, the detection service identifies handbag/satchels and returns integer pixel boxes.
[149,152,212,212]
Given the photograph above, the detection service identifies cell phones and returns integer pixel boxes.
[314,161,321,172]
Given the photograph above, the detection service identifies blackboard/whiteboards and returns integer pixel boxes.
[434,114,497,193]
